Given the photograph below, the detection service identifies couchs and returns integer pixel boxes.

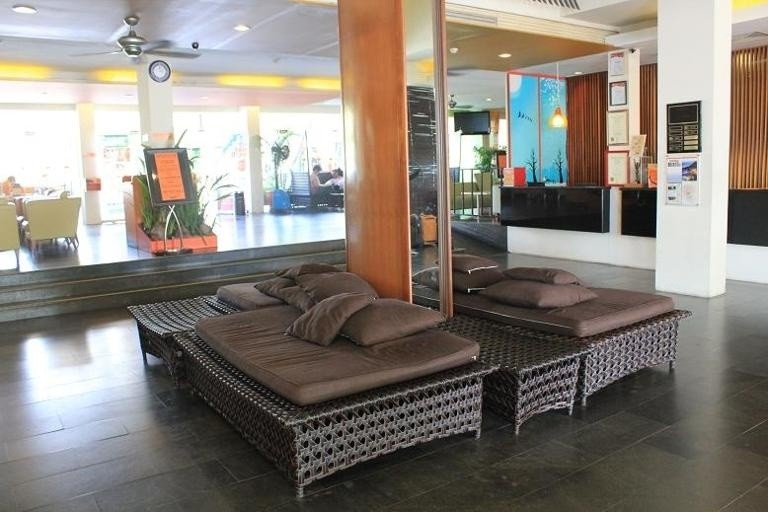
[452,180,494,208]
[293,172,343,209]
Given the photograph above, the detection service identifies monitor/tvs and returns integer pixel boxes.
[453,110,491,135]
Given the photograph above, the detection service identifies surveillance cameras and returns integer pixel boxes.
[629,49,635,53]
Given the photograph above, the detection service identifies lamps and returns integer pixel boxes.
[547,62,569,129]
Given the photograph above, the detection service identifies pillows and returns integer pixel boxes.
[435,253,499,273]
[412,267,502,294]
[477,278,598,309]
[253,262,447,349]
[502,267,582,286]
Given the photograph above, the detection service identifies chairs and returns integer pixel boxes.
[0,190,81,253]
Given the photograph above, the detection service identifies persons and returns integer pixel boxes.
[1,176,24,196]
[310,165,343,209]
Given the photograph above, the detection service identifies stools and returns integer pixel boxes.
[195,283,281,314]
[435,314,595,435]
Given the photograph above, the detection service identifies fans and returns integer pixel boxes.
[67,15,200,60]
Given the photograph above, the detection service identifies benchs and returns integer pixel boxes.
[451,286,694,406]
[174,305,499,499]
[127,296,224,390]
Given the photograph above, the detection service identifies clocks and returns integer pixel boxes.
[149,60,171,83]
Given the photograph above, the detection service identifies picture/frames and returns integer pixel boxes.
[609,81,627,106]
[143,148,196,206]
[604,151,630,188]
[605,109,629,147]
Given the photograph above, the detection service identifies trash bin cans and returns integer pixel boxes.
[235,191,245,216]
